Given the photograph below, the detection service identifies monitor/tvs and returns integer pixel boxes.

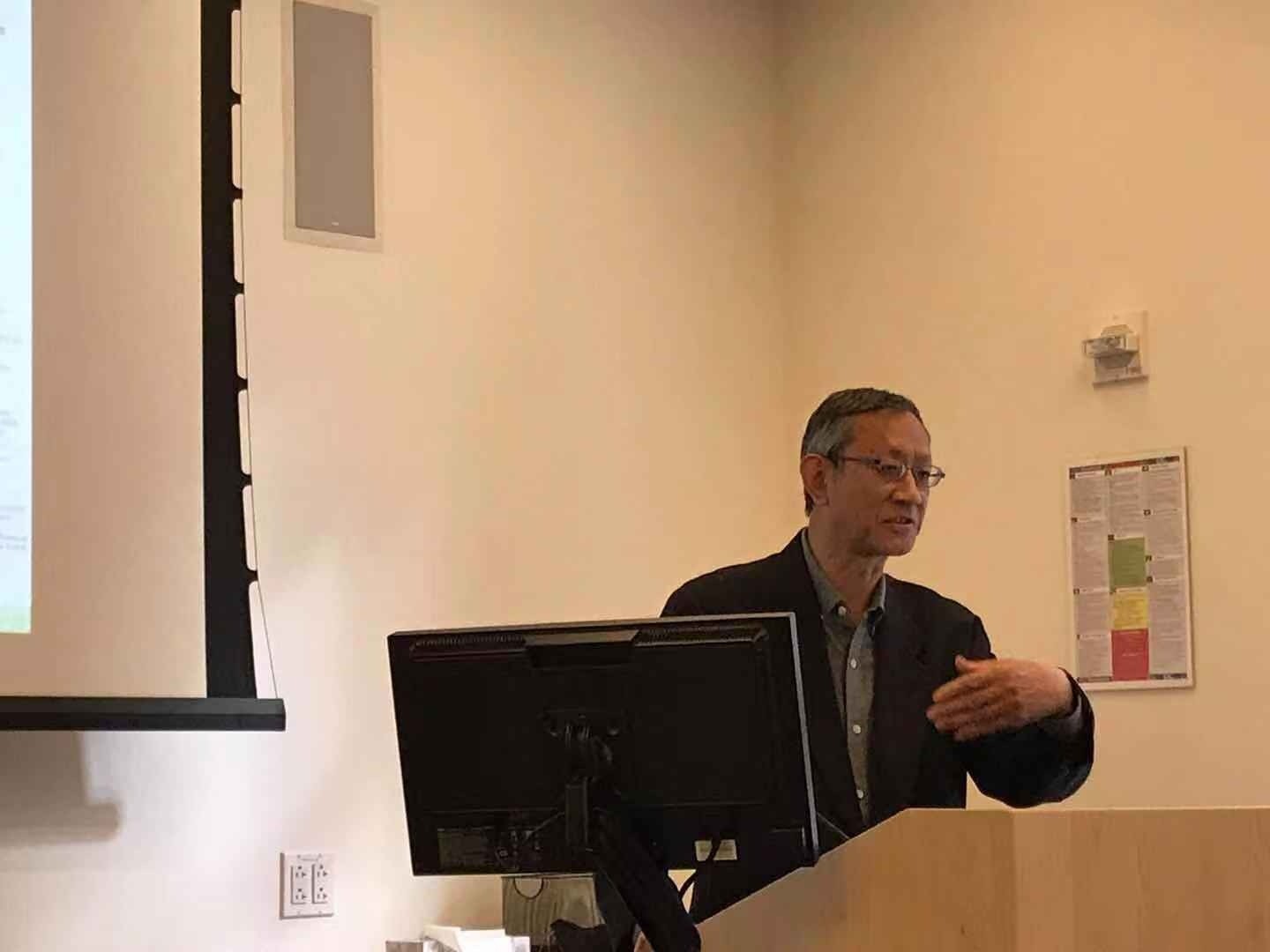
[386,613,816,877]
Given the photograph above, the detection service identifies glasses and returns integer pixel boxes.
[830,452,946,488]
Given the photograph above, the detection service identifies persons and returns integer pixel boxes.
[608,388,1096,952]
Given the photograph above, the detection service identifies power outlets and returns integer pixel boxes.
[278,852,338,919]
[1080,312,1148,386]
